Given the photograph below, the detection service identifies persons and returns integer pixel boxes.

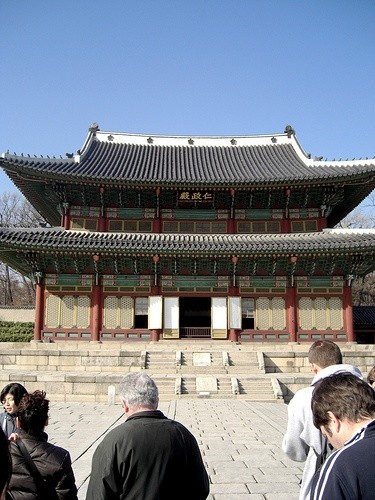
[0,383,80,500]
[282,341,375,500]
[85,371,210,500]
